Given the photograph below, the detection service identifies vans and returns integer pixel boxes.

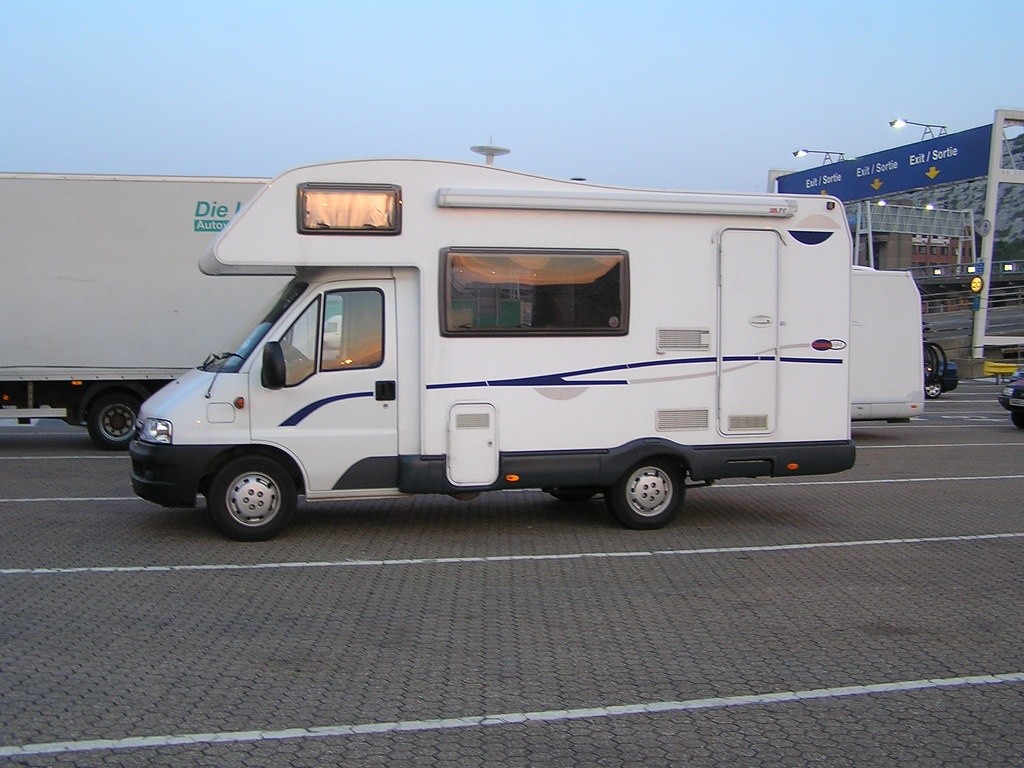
[127,157,859,543]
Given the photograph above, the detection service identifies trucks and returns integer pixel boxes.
[5,171,297,452]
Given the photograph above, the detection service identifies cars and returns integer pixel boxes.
[924,358,960,401]
[998,375,1024,432]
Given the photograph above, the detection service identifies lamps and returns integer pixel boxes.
[792,148,845,162]
[888,118,947,137]
